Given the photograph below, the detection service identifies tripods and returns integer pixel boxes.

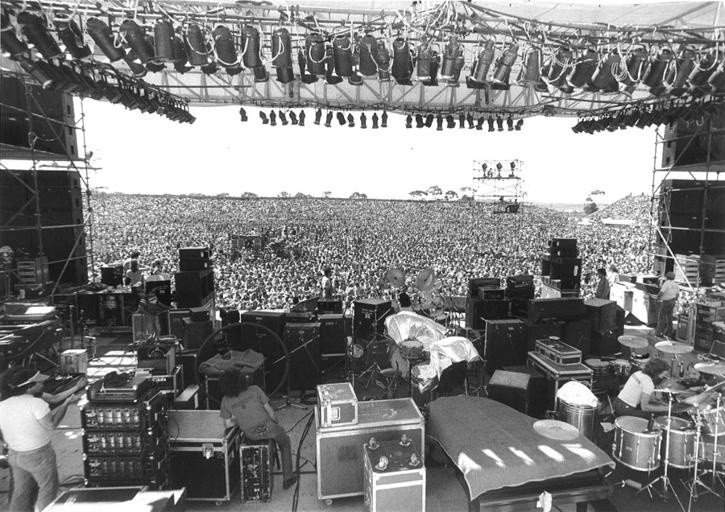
[678,403,724,502]
[684,392,724,489]
[271,335,308,412]
[632,393,681,502]
[361,307,388,389]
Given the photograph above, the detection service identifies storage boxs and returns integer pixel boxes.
[166,409,242,506]
[534,338,582,366]
[363,434,427,511]
[527,351,594,420]
[316,382,359,428]
[314,397,426,506]
[16,12,64,60]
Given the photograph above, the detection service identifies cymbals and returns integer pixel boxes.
[653,339,695,355]
[652,386,692,397]
[696,381,725,393]
[413,268,436,294]
[386,268,406,289]
[693,361,725,378]
[616,334,649,350]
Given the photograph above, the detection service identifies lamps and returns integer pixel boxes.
[643,49,674,88]
[406,115,412,128]
[241,25,261,69]
[377,41,390,84]
[618,51,648,87]
[446,115,456,128]
[359,34,379,77]
[289,110,298,125]
[707,58,725,90]
[183,19,221,77]
[620,84,641,98]
[270,110,277,125]
[534,81,548,92]
[507,117,514,131]
[381,110,388,128]
[298,51,319,84]
[547,50,573,87]
[0,14,31,61]
[416,44,433,82]
[467,112,475,129]
[13,52,196,124]
[239,108,247,121]
[465,39,495,89]
[517,46,544,87]
[276,68,296,84]
[392,38,414,86]
[421,56,441,87]
[476,117,485,130]
[169,36,195,74]
[571,94,725,135]
[347,113,355,127]
[348,52,364,86]
[515,119,523,131]
[591,53,622,88]
[259,111,269,124]
[323,111,333,127]
[325,45,344,86]
[687,53,720,87]
[673,59,695,89]
[119,19,167,74]
[212,24,245,77]
[253,60,270,83]
[565,51,599,88]
[459,112,465,128]
[440,36,459,79]
[333,35,353,77]
[154,20,178,64]
[372,113,378,128]
[52,14,92,59]
[487,115,495,132]
[416,114,424,128]
[305,33,327,76]
[649,57,684,97]
[85,17,126,64]
[620,55,651,98]
[424,114,434,128]
[444,49,466,88]
[497,116,504,131]
[337,112,346,125]
[279,111,288,125]
[558,83,575,94]
[114,37,149,78]
[314,108,322,125]
[436,113,443,131]
[360,113,367,129]
[299,110,305,126]
[491,43,520,91]
[271,28,292,69]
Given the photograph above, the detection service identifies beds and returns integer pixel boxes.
[425,393,616,512]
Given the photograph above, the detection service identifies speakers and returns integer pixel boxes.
[560,258,581,291]
[179,247,210,271]
[241,311,286,393]
[353,299,390,336]
[285,323,321,390]
[465,298,511,330]
[550,239,576,258]
[550,258,560,280]
[175,267,214,307]
[101,265,123,286]
[490,369,531,416]
[541,260,550,275]
[528,298,586,320]
[146,281,171,299]
[478,289,504,301]
[483,320,527,366]
[585,298,616,333]
[468,278,499,297]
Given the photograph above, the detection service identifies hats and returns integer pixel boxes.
[19,371,51,387]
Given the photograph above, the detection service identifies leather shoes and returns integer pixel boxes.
[283,476,296,488]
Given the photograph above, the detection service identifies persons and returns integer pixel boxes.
[134,286,169,341]
[82,190,180,294]
[322,267,332,297]
[219,371,299,489]
[2,367,79,506]
[412,267,595,314]
[318,195,664,265]
[610,261,660,288]
[611,360,680,420]
[332,265,398,315]
[400,287,410,310]
[656,272,680,338]
[175,197,321,313]
[596,269,609,302]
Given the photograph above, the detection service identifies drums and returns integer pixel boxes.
[585,356,609,378]
[687,396,725,439]
[610,358,634,380]
[612,414,663,473]
[654,414,699,471]
[397,337,426,361]
